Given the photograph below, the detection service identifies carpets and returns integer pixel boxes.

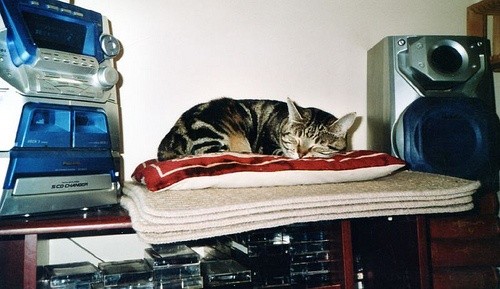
[121,169,480,244]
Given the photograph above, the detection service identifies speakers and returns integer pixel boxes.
[367,36,500,192]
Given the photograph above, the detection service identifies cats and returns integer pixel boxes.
[157,98,357,162]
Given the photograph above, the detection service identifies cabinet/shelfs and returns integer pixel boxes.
[0,182,500,289]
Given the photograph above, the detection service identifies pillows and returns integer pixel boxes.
[131,149,406,193]
